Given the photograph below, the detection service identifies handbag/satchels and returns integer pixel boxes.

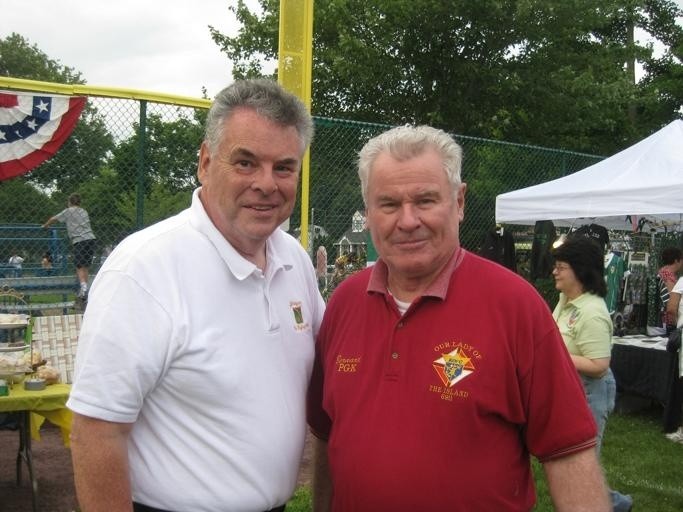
[666,328,682,352]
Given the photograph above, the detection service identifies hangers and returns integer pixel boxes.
[482,222,515,242]
[598,244,626,266]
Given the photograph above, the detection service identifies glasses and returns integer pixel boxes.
[552,264,571,271]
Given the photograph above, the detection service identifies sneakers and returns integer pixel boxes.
[665,426,683,444]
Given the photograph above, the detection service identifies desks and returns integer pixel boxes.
[0,376,78,512]
[609,334,676,424]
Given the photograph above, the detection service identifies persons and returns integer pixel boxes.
[42,251,53,274]
[41,192,97,300]
[334,251,356,276]
[306,123,615,511]
[550,234,637,512]
[8,248,26,278]
[645,246,683,337]
[65,82,329,511]
[665,277,682,444]
